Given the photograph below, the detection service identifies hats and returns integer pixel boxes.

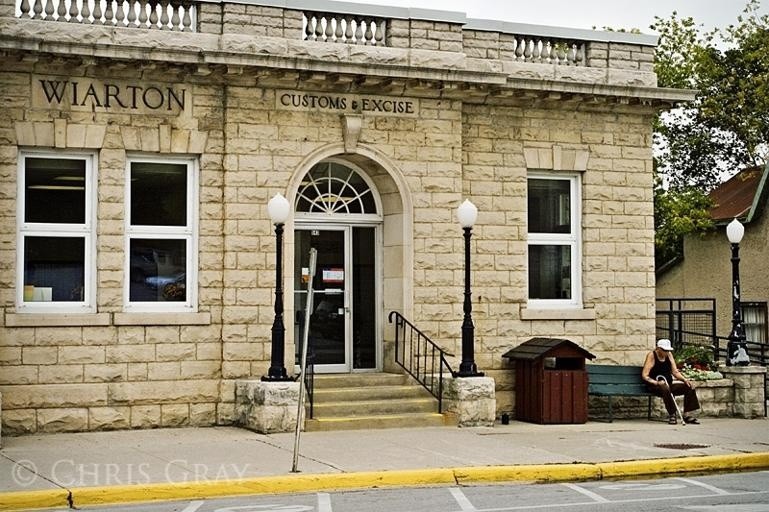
[657,339,675,351]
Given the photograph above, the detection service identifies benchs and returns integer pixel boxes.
[583,363,686,421]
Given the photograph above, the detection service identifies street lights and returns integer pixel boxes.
[454,198,485,376]
[261,193,295,381]
[726,217,750,366]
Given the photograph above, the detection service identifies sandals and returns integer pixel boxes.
[682,416,700,424]
[668,415,677,424]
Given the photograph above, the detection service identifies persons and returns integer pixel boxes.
[640,339,701,424]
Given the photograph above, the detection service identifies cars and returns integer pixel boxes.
[145,272,186,289]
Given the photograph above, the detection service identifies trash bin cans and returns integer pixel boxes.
[501,338,596,424]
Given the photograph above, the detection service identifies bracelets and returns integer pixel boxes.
[656,381,660,385]
